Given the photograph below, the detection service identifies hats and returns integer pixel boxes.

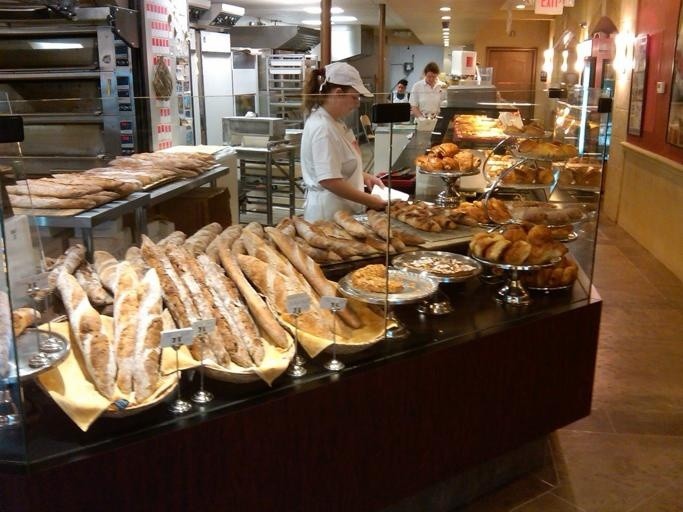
[319,62,374,98]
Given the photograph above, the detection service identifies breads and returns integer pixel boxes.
[6,150,215,208]
[12,308,41,337]
[500,137,602,188]
[386,198,581,286]
[419,142,482,174]
[47,208,426,402]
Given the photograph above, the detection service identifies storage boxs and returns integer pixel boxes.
[451,50,477,76]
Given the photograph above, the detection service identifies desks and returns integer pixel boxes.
[233,145,297,227]
[28,191,151,264]
[143,166,230,209]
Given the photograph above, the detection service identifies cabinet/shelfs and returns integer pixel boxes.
[266,54,318,129]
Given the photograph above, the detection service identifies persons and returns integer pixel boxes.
[409,63,442,125]
[387,80,409,104]
[300,62,385,224]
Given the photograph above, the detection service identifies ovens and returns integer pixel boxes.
[0,5,140,173]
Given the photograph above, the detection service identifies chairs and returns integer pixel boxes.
[360,115,375,173]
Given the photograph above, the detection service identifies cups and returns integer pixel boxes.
[477,66,492,86]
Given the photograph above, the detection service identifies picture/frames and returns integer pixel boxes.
[627,33,648,136]
[665,0,683,149]
